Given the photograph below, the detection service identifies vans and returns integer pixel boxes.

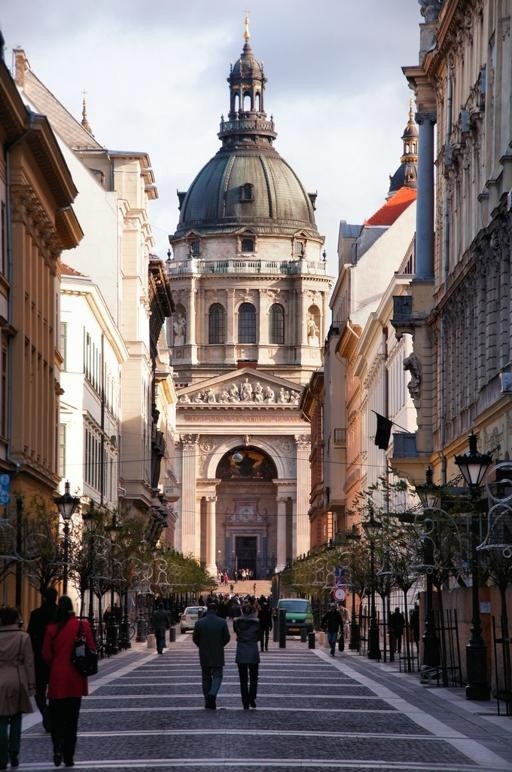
[276,597,314,639]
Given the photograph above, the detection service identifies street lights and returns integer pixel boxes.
[346,522,368,650]
[106,508,123,654]
[138,530,146,641]
[232,551,237,570]
[452,429,498,703]
[153,538,165,562]
[55,480,79,595]
[82,499,100,649]
[414,466,448,671]
[218,550,222,573]
[363,505,387,658]
[121,528,135,648]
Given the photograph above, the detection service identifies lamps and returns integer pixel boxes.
[499,372,511,397]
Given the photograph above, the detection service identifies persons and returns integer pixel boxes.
[151,603,170,654]
[192,603,230,710]
[410,605,419,654]
[114,603,121,624]
[321,603,343,657]
[197,568,273,619]
[42,595,96,767]
[233,605,261,709]
[392,607,405,653]
[179,378,300,405]
[0,606,36,770]
[26,585,59,732]
[257,602,272,652]
[336,603,351,655]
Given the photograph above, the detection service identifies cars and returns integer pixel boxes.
[179,605,208,633]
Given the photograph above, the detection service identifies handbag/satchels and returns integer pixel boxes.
[70,616,100,676]
